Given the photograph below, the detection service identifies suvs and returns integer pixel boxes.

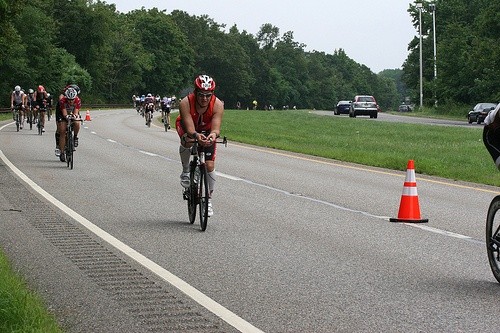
[349,95,378,118]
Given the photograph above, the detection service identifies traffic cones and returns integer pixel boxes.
[83,110,92,121]
[389,159,429,223]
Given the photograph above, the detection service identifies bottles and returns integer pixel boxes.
[193,165,201,183]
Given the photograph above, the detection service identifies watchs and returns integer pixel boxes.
[191,132,198,139]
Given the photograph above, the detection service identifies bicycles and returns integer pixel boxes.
[11,104,54,136]
[134,102,175,133]
[182,135,228,231]
[58,116,84,170]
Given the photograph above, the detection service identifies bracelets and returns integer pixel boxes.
[212,131,218,139]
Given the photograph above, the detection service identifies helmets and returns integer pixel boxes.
[142,95,145,97]
[47,93,50,96]
[15,86,21,91]
[194,75,216,91]
[148,94,151,96]
[38,86,44,91]
[65,88,77,99]
[28,89,34,93]
[163,97,167,100]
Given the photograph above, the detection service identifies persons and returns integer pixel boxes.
[10,84,53,133]
[55,85,82,162]
[176,74,224,216]
[482,102,500,172]
[221,99,297,111]
[131,93,175,129]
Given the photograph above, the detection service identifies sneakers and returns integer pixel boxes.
[203,199,213,216]
[180,171,191,187]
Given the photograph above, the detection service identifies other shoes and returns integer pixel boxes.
[74,138,78,146]
[55,149,60,156]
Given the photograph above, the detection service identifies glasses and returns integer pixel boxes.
[196,92,213,98]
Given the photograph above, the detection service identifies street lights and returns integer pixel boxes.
[417,2,423,109]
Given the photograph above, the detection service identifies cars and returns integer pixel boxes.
[334,100,352,115]
[399,104,415,113]
[467,102,499,125]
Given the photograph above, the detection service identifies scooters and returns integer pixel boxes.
[480,101,500,284]
